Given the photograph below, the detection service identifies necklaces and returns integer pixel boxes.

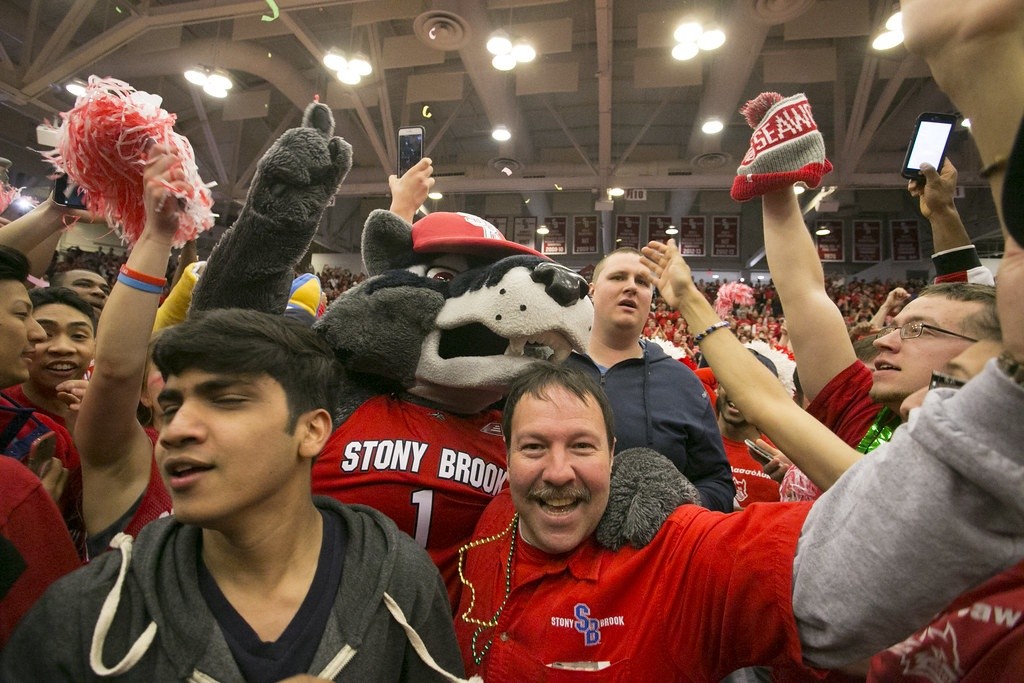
[458,511,523,666]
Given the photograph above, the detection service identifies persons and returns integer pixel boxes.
[317,263,369,308]
[640,0,1024,683]
[0,141,196,683]
[692,349,779,508]
[590,249,738,513]
[1,306,467,682]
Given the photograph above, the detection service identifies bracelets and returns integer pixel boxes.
[998,352,1024,387]
[120,263,167,287]
[693,320,731,348]
[117,272,163,294]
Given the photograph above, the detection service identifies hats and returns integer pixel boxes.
[729,91,834,202]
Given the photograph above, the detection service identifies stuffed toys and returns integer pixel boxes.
[186,102,699,623]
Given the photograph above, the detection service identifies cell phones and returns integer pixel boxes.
[52,173,87,210]
[27,431,57,478]
[397,126,425,179]
[744,439,785,468]
[901,112,957,181]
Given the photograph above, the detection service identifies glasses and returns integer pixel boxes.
[927,369,965,390]
[876,321,979,342]
